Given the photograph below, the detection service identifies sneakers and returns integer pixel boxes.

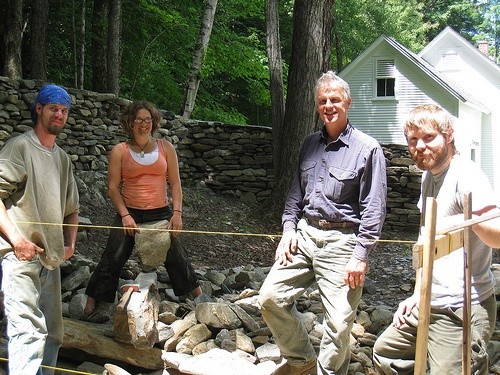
[79,308,109,323]
[272,357,317,375]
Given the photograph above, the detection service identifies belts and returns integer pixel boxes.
[307,218,353,228]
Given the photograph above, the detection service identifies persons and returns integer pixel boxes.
[373,104,500,375]
[78,100,213,324]
[257,70,387,375]
[0,84,81,375]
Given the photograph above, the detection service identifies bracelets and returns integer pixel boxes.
[122,214,130,218]
[173,209,182,213]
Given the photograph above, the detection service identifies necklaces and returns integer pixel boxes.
[134,137,150,158]
